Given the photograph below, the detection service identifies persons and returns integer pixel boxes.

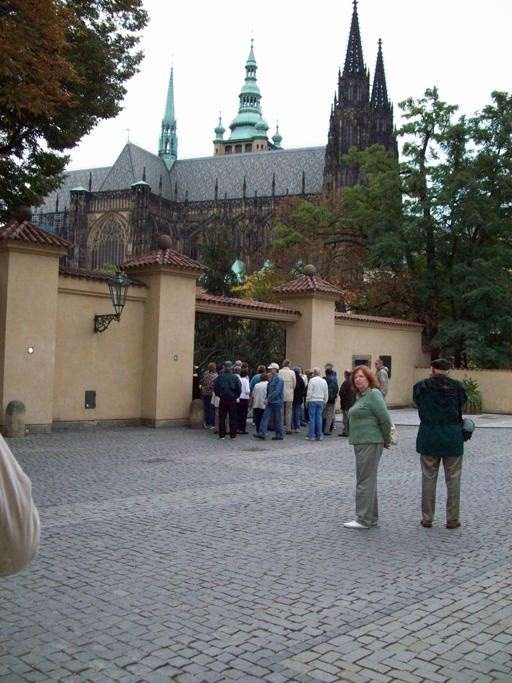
[200,358,389,441]
[413,359,468,528]
[344,365,392,528]
[0,433,40,577]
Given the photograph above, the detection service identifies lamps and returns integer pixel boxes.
[94,270,130,332]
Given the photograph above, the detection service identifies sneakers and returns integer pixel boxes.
[252,422,307,440]
[344,521,377,529]
[204,421,248,440]
[446,520,461,528]
[305,426,348,440]
[420,519,432,527]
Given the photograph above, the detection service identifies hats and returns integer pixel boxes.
[267,362,279,369]
[222,361,234,370]
[429,359,451,370]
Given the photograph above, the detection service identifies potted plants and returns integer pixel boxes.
[459,374,482,415]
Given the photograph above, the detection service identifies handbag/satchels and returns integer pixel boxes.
[390,425,397,445]
[463,418,475,441]
[211,392,220,408]
